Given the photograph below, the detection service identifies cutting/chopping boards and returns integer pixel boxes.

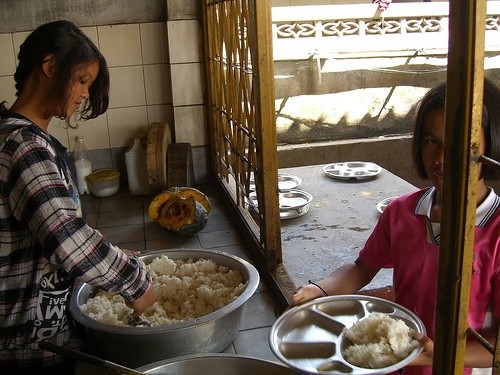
[168,142,196,191]
[146,122,171,195]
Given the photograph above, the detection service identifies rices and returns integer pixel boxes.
[79,254,249,328]
[342,311,419,368]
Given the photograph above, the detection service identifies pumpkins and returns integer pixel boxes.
[149,186,211,235]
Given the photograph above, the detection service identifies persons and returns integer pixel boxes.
[291,77,500,375]
[0,19,158,375]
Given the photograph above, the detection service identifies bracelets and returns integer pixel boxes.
[308,279,329,297]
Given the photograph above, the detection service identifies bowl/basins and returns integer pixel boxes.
[72,136,92,197]
[119,353,303,375]
[68,248,260,365]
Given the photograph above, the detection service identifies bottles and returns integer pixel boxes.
[124,137,150,196]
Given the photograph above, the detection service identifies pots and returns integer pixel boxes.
[87,166,120,197]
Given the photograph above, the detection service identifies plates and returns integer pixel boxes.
[377,196,405,214]
[269,295,426,371]
[322,161,383,182]
[247,170,302,190]
[244,190,313,219]
